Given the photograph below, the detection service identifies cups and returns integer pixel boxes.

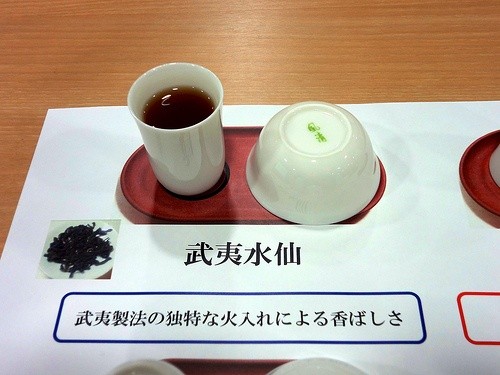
[127,62,225,195]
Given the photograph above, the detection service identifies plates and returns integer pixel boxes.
[120,126,386,221]
[459,129,500,217]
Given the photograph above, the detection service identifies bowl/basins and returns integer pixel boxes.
[246,102,380,225]
